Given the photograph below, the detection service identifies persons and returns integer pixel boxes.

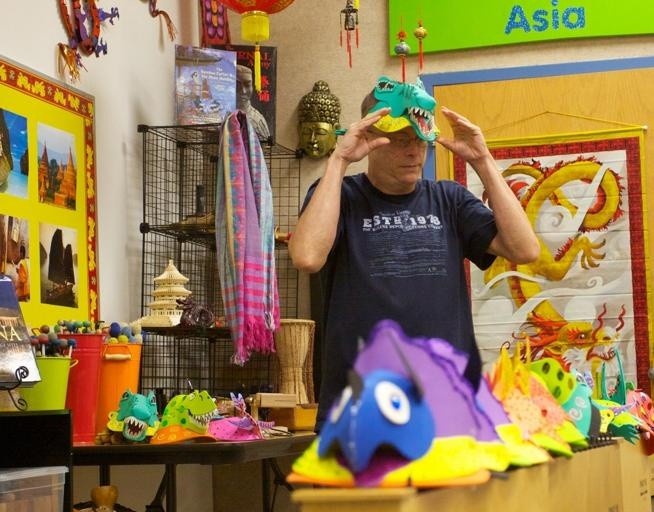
[236,65,270,142]
[297,80,341,158]
[15,245,30,302]
[287,87,541,434]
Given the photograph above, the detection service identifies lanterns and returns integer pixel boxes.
[216,0,294,43]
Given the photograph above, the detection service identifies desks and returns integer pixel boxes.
[72,431,317,512]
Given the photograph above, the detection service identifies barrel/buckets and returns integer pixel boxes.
[19,356,80,411]
[95,342,141,432]
[56,334,104,443]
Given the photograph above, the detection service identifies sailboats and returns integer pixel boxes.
[44,227,76,301]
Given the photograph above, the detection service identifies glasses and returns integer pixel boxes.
[367,128,427,147]
